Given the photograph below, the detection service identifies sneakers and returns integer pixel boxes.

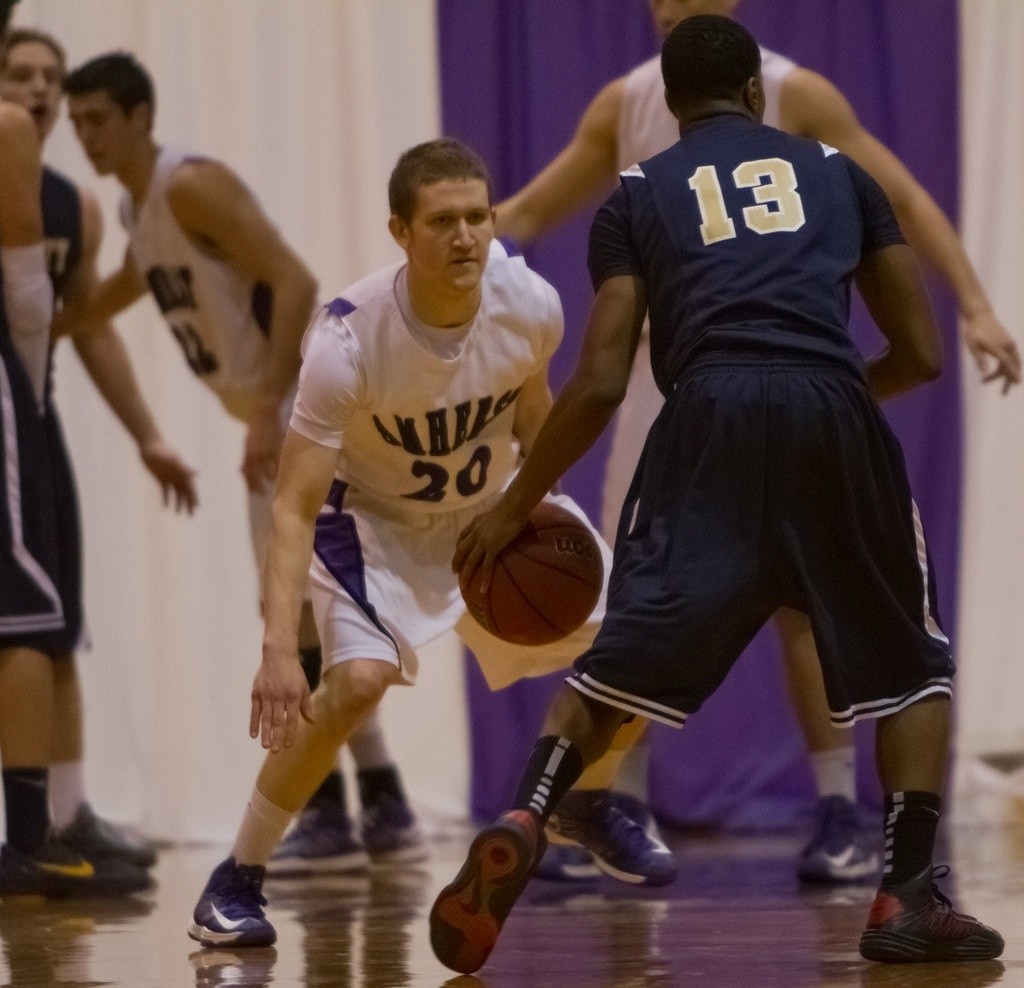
[187,948,277,988]
[0,822,155,895]
[859,863,1005,962]
[187,856,277,947]
[798,794,880,882]
[265,804,371,874]
[429,810,547,974]
[352,800,429,865]
[536,794,659,880]
[543,791,680,886]
[53,801,155,865]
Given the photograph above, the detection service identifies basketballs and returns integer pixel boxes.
[457,500,605,647]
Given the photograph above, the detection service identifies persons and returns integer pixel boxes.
[428,8,1011,974]
[0,0,1023,903]
[185,135,684,952]
